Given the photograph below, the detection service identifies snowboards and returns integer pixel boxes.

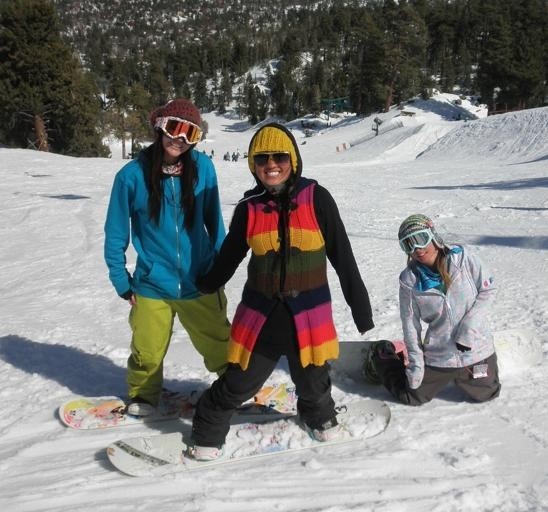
[58,382,298,429]
[327,340,542,387]
[107,399,391,477]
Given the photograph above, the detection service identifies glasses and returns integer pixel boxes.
[154,116,203,144]
[398,228,434,254]
[253,152,290,166]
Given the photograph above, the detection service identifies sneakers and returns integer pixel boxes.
[194,447,218,460]
[364,340,396,385]
[128,398,154,416]
[297,413,340,441]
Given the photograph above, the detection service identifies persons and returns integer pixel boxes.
[373,214,508,406]
[185,123,372,457]
[105,97,255,417]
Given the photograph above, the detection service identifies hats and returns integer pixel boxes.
[398,214,444,249]
[151,99,208,139]
[249,127,297,174]
